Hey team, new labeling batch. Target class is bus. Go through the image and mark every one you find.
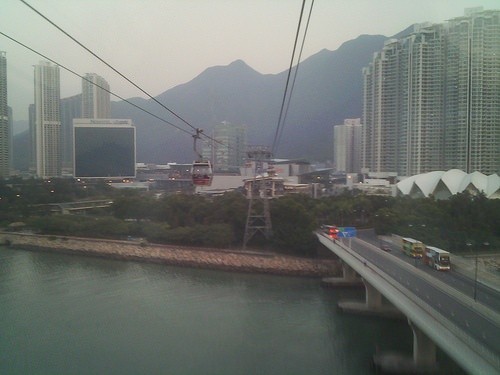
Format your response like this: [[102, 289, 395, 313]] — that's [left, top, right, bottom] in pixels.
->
[[424, 245, 451, 271], [321, 223, 390, 252], [403, 238, 424, 259]]
